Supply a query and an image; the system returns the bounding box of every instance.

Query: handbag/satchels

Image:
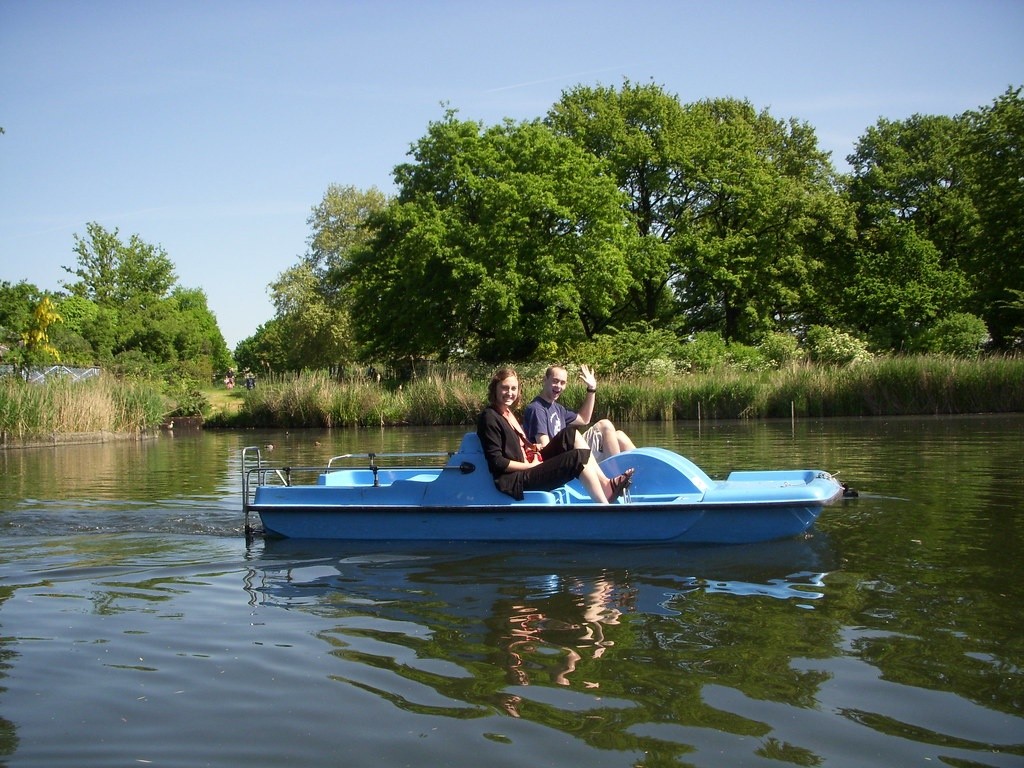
[524,448,542,463]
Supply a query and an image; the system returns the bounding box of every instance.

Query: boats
[239,433,849,544]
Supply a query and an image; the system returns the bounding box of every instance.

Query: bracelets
[586,387,596,392]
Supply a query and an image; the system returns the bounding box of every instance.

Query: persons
[477,368,635,503]
[225,367,235,389]
[523,364,636,459]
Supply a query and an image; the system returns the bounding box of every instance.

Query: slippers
[608,467,634,503]
